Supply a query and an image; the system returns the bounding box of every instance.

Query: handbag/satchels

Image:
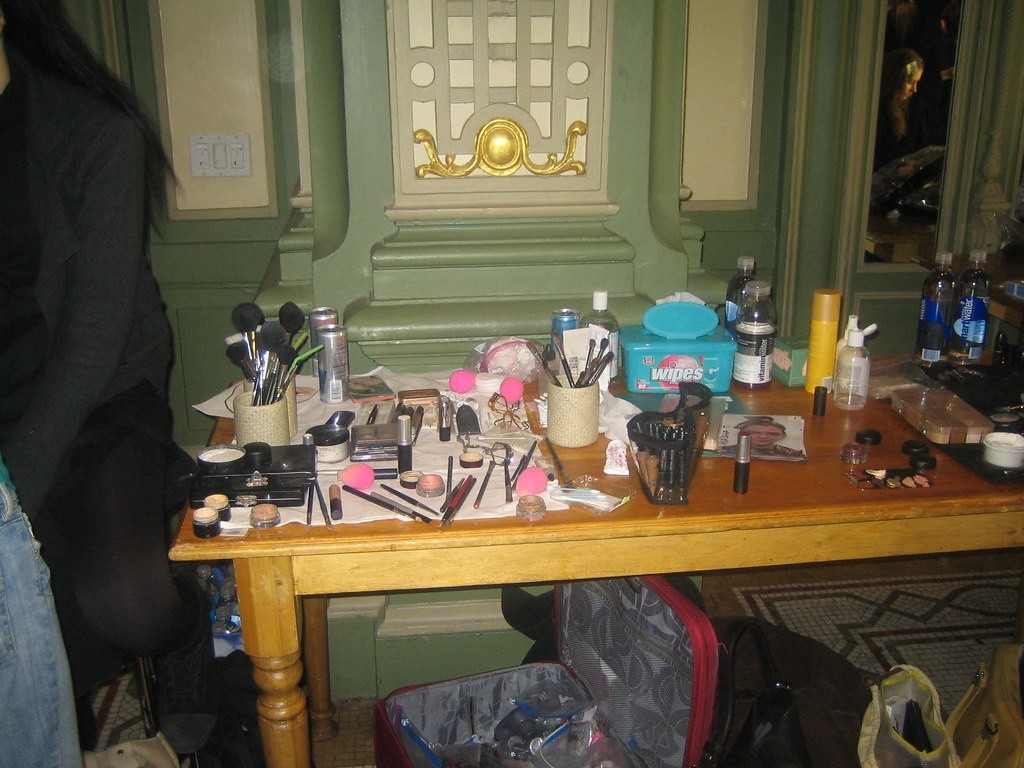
[946,643,1024,768]
[708,586,878,768]
[857,664,961,768]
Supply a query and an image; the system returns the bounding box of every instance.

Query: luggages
[372,575,720,768]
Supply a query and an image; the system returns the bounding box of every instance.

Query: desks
[168,370,1023,768]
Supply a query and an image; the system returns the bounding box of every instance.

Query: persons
[873,48,924,173]
[0,0,217,755]
[721,416,806,458]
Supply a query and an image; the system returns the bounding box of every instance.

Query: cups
[548,375,599,448]
[233,374,298,447]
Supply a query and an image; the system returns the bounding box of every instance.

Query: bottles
[835,315,860,390]
[726,256,776,393]
[833,322,877,411]
[912,248,990,368]
[579,290,620,384]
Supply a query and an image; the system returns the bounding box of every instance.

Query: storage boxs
[984,255,1024,313]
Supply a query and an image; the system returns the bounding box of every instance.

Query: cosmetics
[189,288,1024,538]
[431,676,632,768]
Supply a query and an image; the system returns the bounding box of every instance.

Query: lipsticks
[734,430,752,497]
